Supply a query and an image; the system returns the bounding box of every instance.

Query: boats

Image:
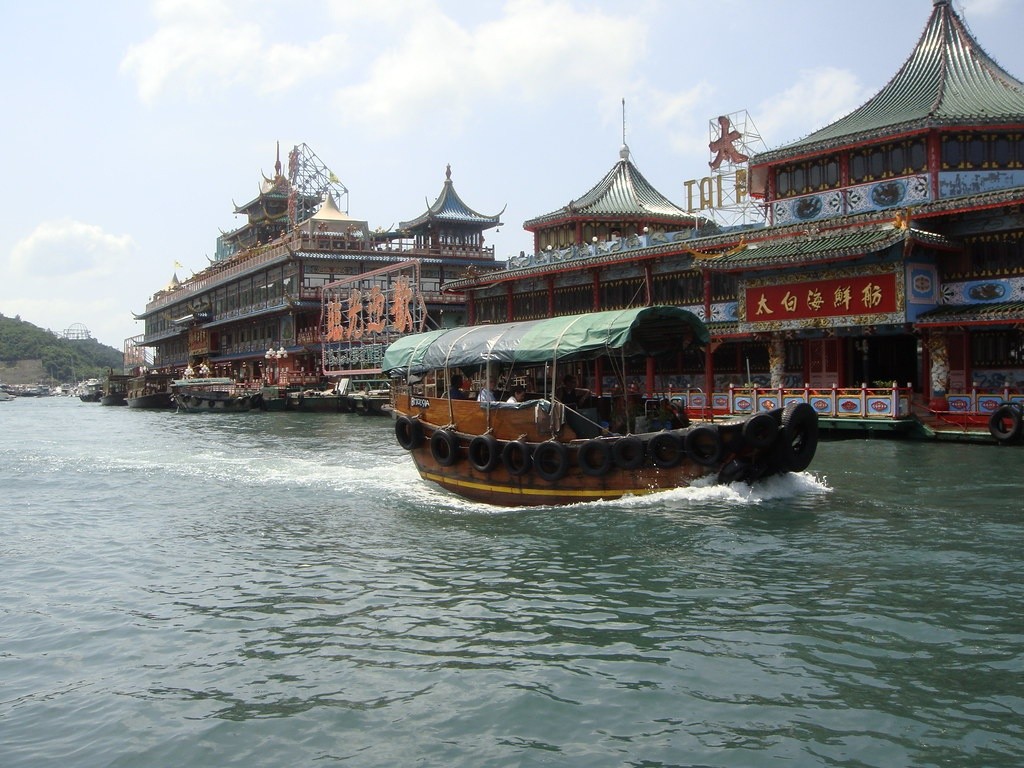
[97,373,139,406]
[381,301,820,508]
[167,375,264,415]
[334,379,392,416]
[283,367,383,412]
[0,358,104,403]
[122,373,180,408]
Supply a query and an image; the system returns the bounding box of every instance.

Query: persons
[450,375,466,399]
[506,386,526,402]
[477,376,496,401]
[557,375,590,410]
[437,374,447,397]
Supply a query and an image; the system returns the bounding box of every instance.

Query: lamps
[592,237,598,242]
[547,245,552,250]
[644,227,648,233]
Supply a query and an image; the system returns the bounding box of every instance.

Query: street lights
[276,346,289,382]
[264,348,277,385]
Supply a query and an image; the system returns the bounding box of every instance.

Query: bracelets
[581,397,588,402]
